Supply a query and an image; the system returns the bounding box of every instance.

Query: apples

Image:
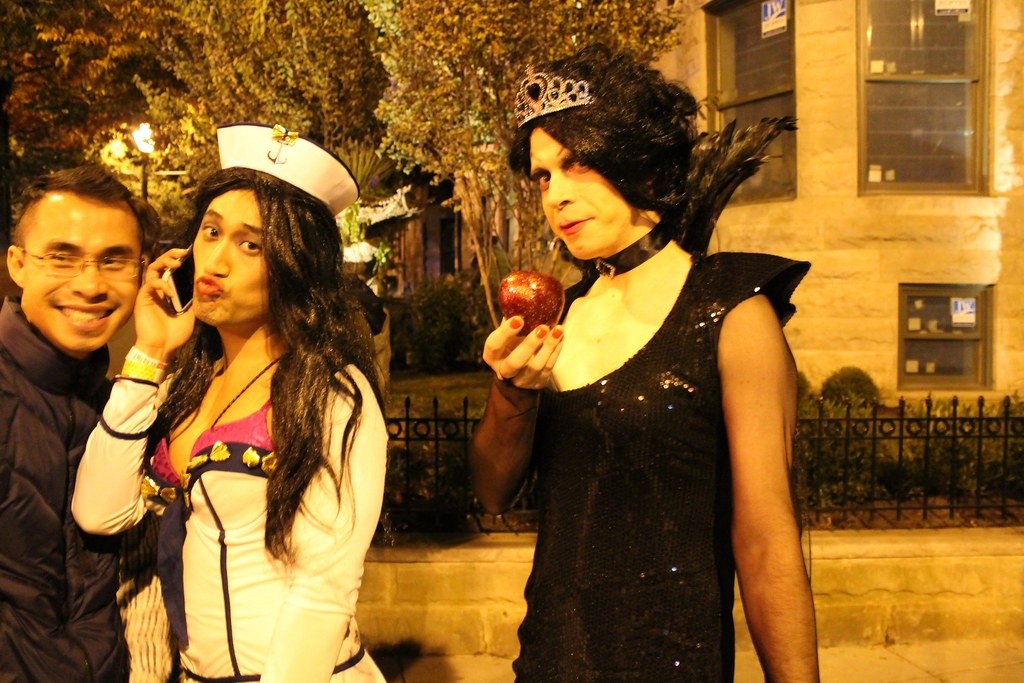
[498,270,565,337]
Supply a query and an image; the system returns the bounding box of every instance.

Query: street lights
[133,123,156,202]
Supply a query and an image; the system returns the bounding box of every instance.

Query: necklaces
[211,352,284,428]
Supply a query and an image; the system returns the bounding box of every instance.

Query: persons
[469,44,820,683]
[71,123,390,683]
[0,164,162,683]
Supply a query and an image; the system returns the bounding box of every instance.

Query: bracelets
[125,348,167,384]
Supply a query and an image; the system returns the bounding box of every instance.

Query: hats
[216,122,360,219]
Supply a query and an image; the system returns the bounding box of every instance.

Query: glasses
[24,251,142,280]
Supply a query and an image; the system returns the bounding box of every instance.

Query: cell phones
[162,244,196,312]
